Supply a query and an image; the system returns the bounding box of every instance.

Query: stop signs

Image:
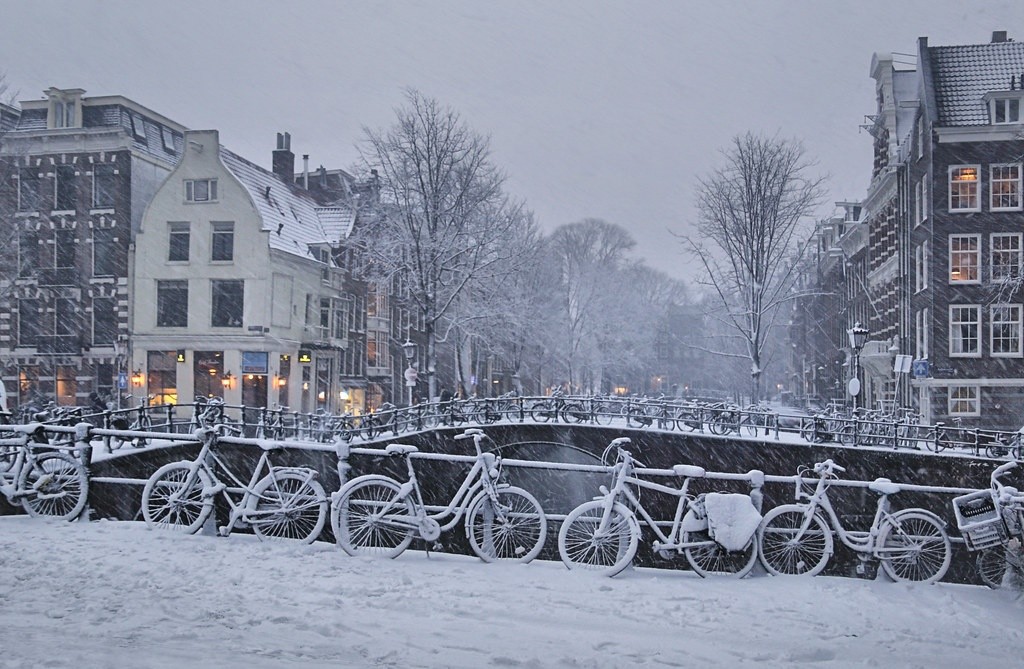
[405,368,418,382]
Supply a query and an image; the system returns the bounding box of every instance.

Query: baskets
[952,489,1010,551]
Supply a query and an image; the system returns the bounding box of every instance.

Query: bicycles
[952,461,1024,589]
[0,424,90,522]
[440,386,1024,460]
[0,391,442,453]
[142,398,333,545]
[757,459,953,585]
[330,429,547,563]
[557,436,760,578]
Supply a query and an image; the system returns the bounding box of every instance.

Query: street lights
[113,334,129,416]
[402,339,418,409]
[847,321,871,447]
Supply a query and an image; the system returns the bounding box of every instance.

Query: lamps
[401,338,418,360]
[846,322,870,352]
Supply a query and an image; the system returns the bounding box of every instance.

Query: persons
[437,381,466,412]
